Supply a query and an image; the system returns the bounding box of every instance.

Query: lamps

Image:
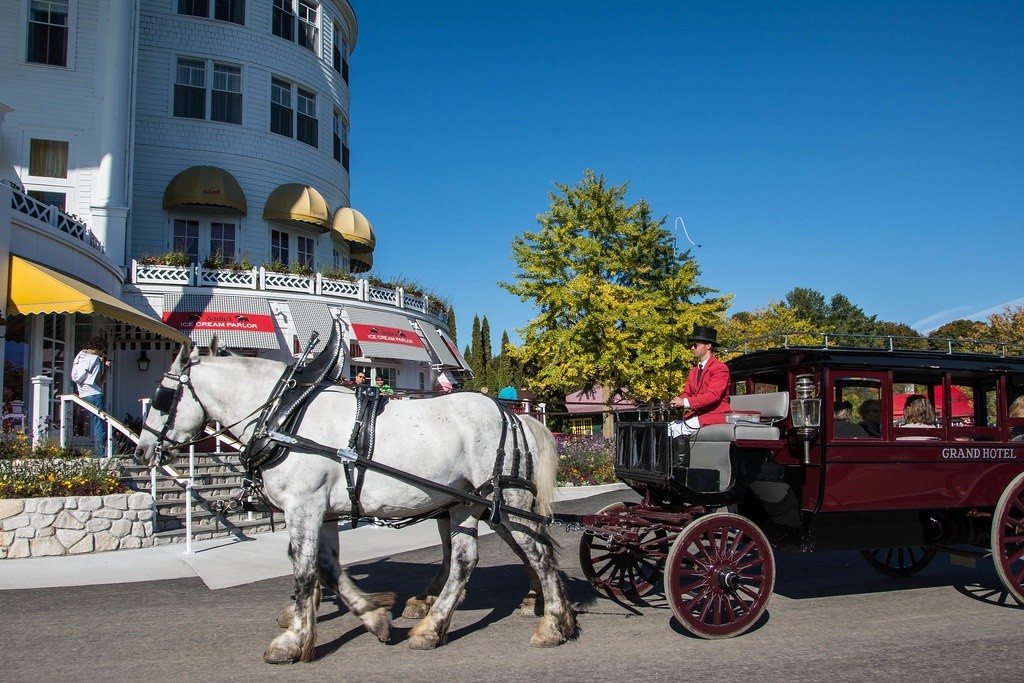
[136,350,151,371]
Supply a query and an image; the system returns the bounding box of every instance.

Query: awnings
[6,253,192,353]
[263,183,333,233]
[350,245,373,273]
[329,206,375,249]
[163,165,248,215]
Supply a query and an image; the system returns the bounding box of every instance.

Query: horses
[134,333,581,667]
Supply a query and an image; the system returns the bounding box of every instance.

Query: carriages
[131,326,1024,663]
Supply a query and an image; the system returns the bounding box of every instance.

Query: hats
[687,327,721,346]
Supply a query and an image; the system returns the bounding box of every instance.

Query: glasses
[359,376,365,379]
[690,343,707,349]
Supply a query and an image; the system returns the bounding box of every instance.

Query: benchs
[678,390,789,490]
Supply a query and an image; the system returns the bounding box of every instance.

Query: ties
[697,364,703,386]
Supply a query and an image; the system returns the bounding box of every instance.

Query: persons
[2,384,25,432]
[373,374,395,395]
[1007,394,1024,442]
[349,372,366,390]
[70,335,112,467]
[895,395,940,440]
[833,398,869,438]
[666,326,732,440]
[857,398,881,437]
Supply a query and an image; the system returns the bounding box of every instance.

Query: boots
[673,434,690,468]
